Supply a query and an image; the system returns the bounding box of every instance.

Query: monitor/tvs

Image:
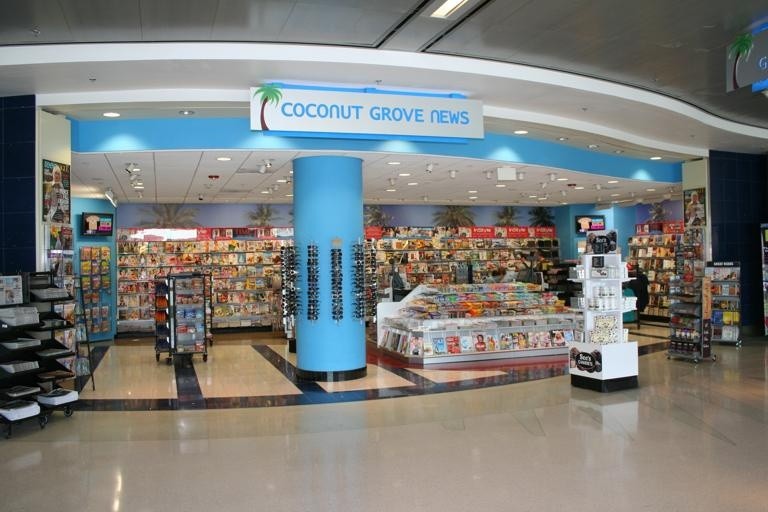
[574,215,606,234]
[82,212,113,236]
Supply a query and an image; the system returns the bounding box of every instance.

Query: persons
[685,190,706,226]
[44,164,66,222]
[482,267,507,284]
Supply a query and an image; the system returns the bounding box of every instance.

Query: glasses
[280,245,298,317]
[307,245,320,320]
[351,243,377,318]
[330,248,344,320]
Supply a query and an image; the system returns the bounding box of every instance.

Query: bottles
[569,264,621,312]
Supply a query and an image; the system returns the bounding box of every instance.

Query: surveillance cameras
[199,195,203,200]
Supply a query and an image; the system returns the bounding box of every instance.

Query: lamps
[125,163,145,200]
[548,173,556,183]
[105,186,118,210]
[259,159,273,175]
[485,171,491,180]
[450,171,457,179]
[518,171,525,182]
[425,163,435,174]
[595,184,601,191]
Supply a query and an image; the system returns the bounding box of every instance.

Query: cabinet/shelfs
[114,225,292,354]
[364,225,581,313]
[572,234,738,382]
[0,272,82,425]
